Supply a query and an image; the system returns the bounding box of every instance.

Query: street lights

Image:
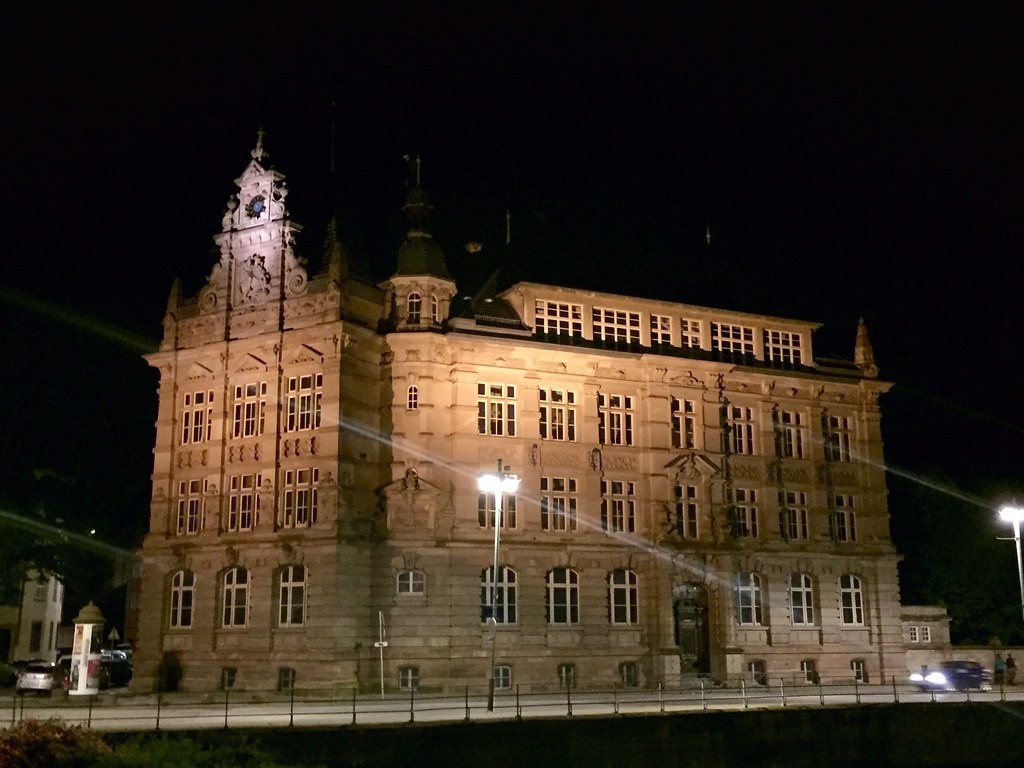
[996,505,1024,625]
[475,474,519,712]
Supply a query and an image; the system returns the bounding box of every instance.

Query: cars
[910,661,992,693]
[0,643,133,688]
[16,662,56,696]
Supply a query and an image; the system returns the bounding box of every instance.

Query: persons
[994,654,1016,684]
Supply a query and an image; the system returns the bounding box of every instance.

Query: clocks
[249,195,266,218]
